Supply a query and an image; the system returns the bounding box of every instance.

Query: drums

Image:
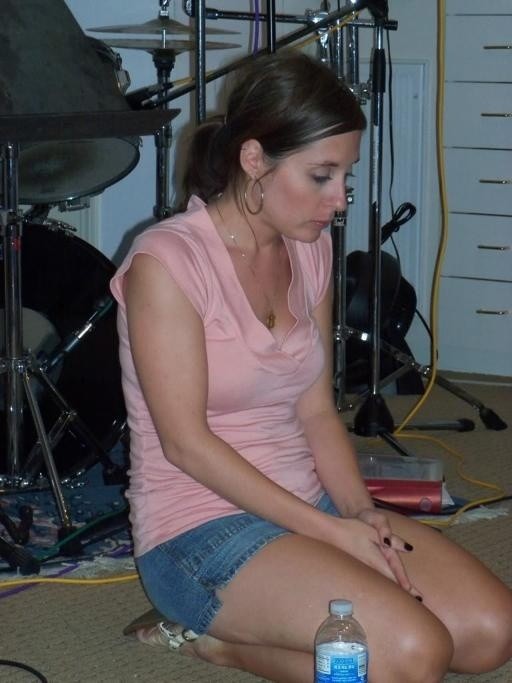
[0,221,127,494]
[0,1,140,205]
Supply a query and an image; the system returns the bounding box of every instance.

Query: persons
[106,40,510,683]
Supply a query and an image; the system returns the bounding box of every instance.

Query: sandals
[122,608,200,651]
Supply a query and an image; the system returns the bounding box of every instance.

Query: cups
[363,478,443,516]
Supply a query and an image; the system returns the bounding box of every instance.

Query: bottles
[314,599,369,683]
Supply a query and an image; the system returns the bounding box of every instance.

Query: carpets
[0,419,509,580]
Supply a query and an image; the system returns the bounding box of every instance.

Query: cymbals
[99,40,243,57]
[85,16,242,34]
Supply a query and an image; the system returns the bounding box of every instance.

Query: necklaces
[215,195,288,329]
